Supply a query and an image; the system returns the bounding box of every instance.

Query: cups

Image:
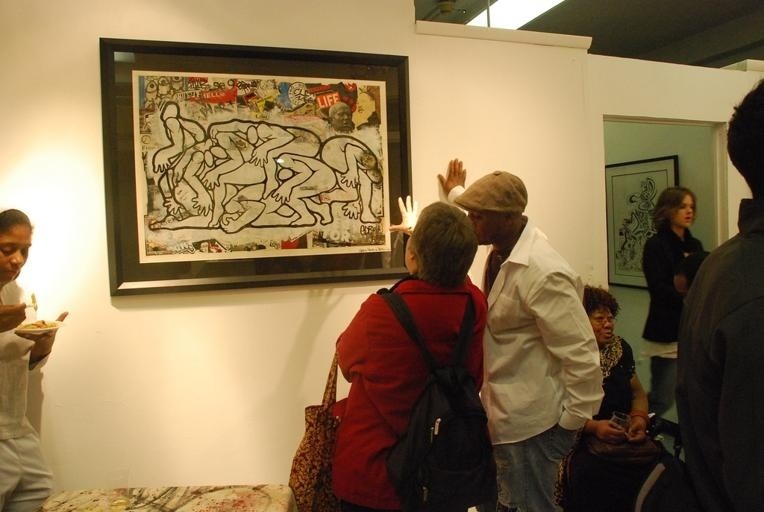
[609,411,633,432]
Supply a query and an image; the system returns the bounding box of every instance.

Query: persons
[575,284,659,468]
[675,80,764,512]
[332,197,489,512]
[641,187,710,416]
[329,102,352,131]
[0,209,69,512]
[437,159,605,512]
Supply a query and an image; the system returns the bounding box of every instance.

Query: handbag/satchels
[633,453,700,512]
[583,411,658,464]
[288,399,343,511]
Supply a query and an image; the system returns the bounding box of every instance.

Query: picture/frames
[605,153,680,290]
[98,36,413,298]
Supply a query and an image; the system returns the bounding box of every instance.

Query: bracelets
[630,410,648,421]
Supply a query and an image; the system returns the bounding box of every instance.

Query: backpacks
[385,292,498,511]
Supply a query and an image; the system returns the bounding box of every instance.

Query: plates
[16,321,63,334]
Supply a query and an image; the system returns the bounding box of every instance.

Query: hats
[454,171,528,214]
